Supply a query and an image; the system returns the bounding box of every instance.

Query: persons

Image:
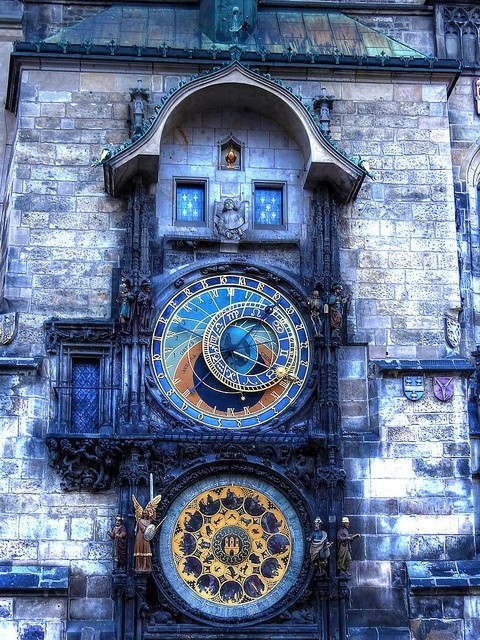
[337,516,354,576]
[217,198,247,240]
[115,278,136,334]
[132,508,155,575]
[135,279,155,336]
[328,284,350,339]
[305,515,333,576]
[106,516,129,573]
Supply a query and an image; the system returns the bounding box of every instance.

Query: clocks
[143,267,316,433]
